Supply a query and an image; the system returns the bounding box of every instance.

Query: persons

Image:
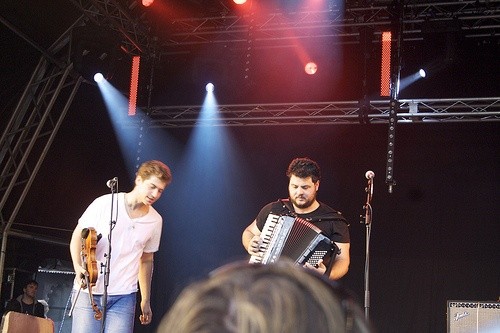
[70,160,172,332]
[0,256,375,333]
[242,158,350,279]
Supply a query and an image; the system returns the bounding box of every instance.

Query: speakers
[446,300,500,333]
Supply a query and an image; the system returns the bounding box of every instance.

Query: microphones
[106,177,119,188]
[365,170,375,179]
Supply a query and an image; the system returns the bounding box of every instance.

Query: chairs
[1,311,54,333]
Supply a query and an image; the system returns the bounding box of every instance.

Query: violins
[80,227,103,321]
